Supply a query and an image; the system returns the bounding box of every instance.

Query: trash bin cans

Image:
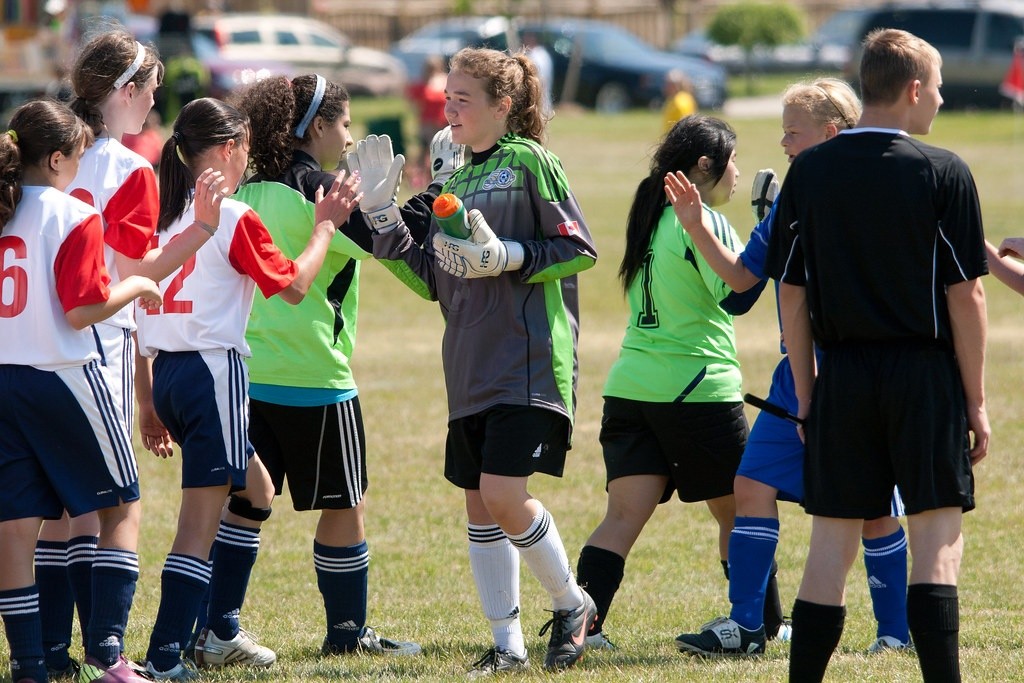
[366,113,406,161]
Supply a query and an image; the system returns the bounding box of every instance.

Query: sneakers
[144,658,198,683]
[674,616,767,660]
[537,587,598,672]
[585,629,620,652]
[203,627,275,667]
[182,632,198,670]
[869,633,913,655]
[316,624,419,663]
[774,614,794,641]
[46,655,81,682]
[194,628,209,669]
[77,655,153,683]
[467,645,530,680]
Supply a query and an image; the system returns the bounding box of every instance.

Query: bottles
[432,194,472,240]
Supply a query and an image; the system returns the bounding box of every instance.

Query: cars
[385,15,511,78]
[62,5,294,119]
[671,4,873,77]
[194,10,406,99]
[441,15,730,115]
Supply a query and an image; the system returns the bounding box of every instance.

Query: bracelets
[195,219,217,236]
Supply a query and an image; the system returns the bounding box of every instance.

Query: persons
[763,27,991,683]
[346,48,598,672]
[200,74,469,669]
[663,77,914,660]
[0,98,164,683]
[11,28,165,680]
[524,27,552,124]
[576,117,792,652]
[134,98,367,683]
[985,237,1024,296]
[663,72,695,138]
[405,54,454,187]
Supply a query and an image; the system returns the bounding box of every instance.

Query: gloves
[345,134,415,253]
[432,209,525,278]
[749,169,779,225]
[428,125,466,187]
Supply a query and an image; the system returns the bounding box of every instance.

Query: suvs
[840,0,1024,111]
